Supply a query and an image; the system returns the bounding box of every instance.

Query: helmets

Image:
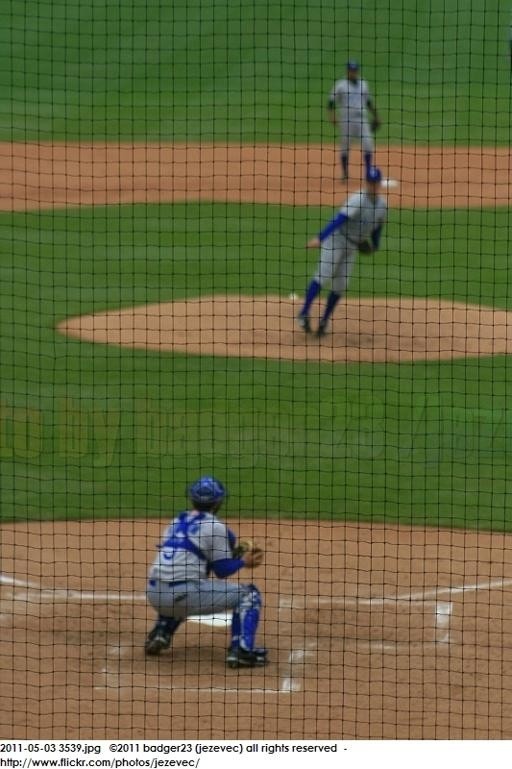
[191,475,225,503]
[366,166,381,181]
[347,62,358,71]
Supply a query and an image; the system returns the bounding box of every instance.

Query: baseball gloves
[235,536,266,567]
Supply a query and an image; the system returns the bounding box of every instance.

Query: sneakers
[317,320,327,332]
[226,647,268,666]
[298,312,310,330]
[145,630,171,654]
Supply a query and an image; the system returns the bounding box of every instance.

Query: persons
[327,62,381,182]
[144,476,269,669]
[297,167,389,337]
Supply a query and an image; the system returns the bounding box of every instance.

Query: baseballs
[290,293,299,300]
